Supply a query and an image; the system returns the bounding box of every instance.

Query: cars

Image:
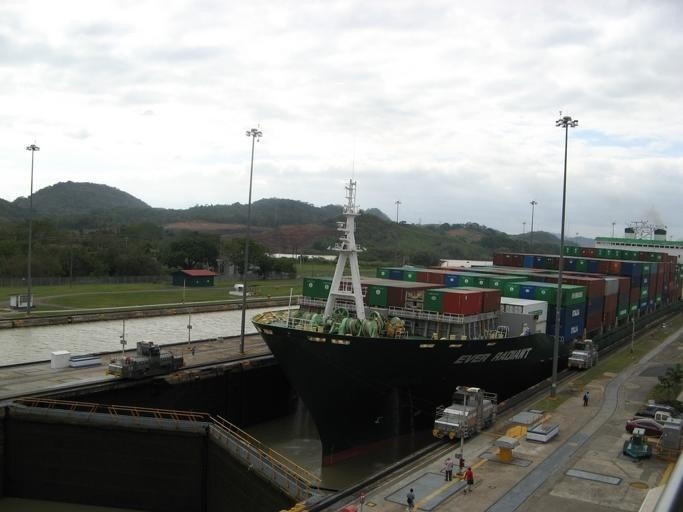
[625,418,663,437]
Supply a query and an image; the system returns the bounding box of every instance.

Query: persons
[445,458,453,481]
[406,488,415,512]
[463,467,473,493]
[520,323,529,336]
[583,392,591,406]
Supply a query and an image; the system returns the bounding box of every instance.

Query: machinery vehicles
[106,340,187,381]
[429,384,497,440]
[568,337,598,369]
[622,428,652,460]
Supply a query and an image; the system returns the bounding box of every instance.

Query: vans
[638,404,678,417]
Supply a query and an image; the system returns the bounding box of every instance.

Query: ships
[252,179,683,464]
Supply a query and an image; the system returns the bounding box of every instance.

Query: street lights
[394,200,405,224]
[549,113,579,400]
[524,195,617,242]
[240,128,262,355]
[26,145,39,316]
[359,492,365,512]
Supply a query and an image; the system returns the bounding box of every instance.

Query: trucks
[629,411,682,429]
